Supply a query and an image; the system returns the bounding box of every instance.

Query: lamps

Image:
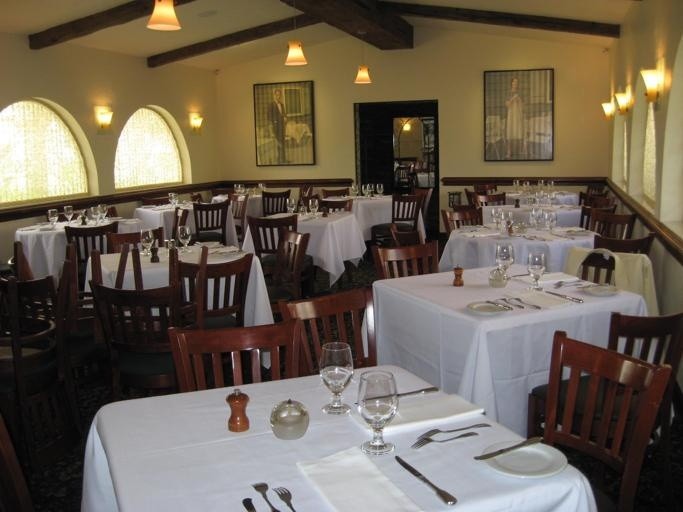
[598,69,665,120]
[354,40,372,85]
[284,2,307,66]
[144,1,181,32]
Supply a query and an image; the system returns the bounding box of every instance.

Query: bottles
[452,264,464,286]
[487,266,508,286]
[270,396,309,441]
[224,388,249,432]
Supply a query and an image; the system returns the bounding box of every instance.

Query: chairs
[396,166,411,194]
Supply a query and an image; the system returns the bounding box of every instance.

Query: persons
[503,75,525,159]
[265,87,294,164]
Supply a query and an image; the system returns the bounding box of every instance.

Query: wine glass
[487,178,557,238]
[47,183,385,262]
[527,254,546,292]
[496,244,514,280]
[359,369,400,456]
[319,342,354,416]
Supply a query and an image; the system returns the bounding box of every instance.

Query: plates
[584,284,621,297]
[481,440,567,478]
[466,301,501,315]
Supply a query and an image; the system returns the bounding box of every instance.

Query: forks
[253,480,281,512]
[416,422,491,440]
[411,432,478,450]
[271,486,296,512]
[501,296,543,311]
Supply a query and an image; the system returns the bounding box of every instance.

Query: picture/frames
[252,80,315,167]
[482,67,553,162]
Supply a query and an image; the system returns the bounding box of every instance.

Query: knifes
[394,455,456,504]
[487,300,511,310]
[242,498,255,512]
[354,386,438,404]
[545,291,583,303]
[473,436,542,460]
